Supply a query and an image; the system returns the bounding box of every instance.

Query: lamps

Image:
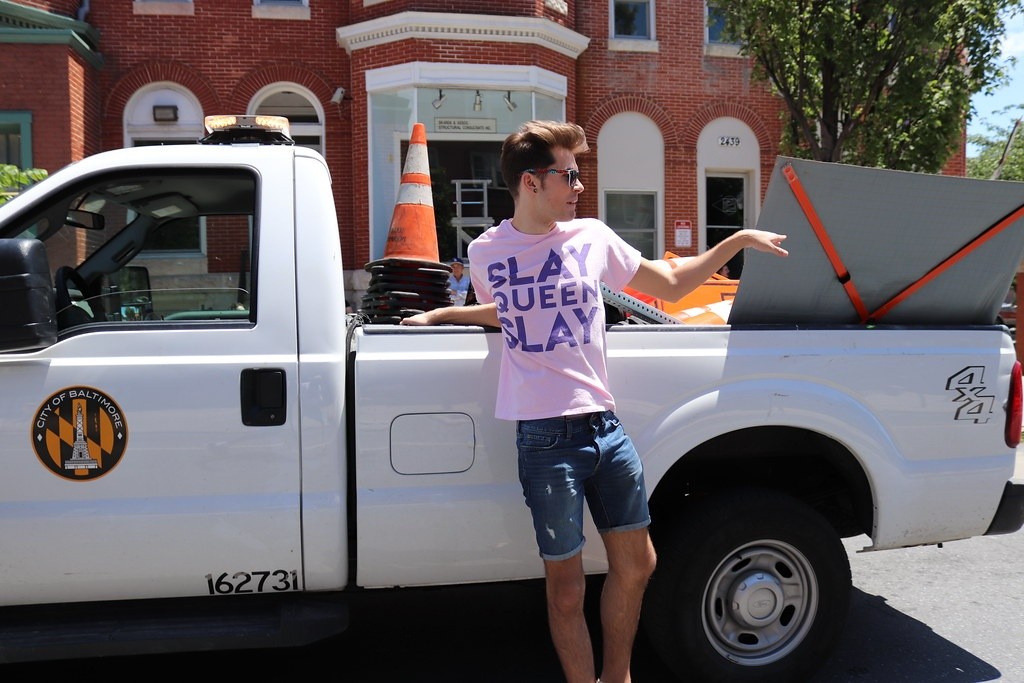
[504,90,520,110]
[472,89,483,112]
[430,89,449,110]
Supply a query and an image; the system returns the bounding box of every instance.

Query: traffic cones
[358,120,454,335]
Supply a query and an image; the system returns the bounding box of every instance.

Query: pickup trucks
[0,114,1021,682]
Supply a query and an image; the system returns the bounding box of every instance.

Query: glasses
[517,167,580,188]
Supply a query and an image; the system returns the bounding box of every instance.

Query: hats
[451,258,465,266]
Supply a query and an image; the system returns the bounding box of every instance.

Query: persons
[447,257,470,298]
[399,121,790,683]
